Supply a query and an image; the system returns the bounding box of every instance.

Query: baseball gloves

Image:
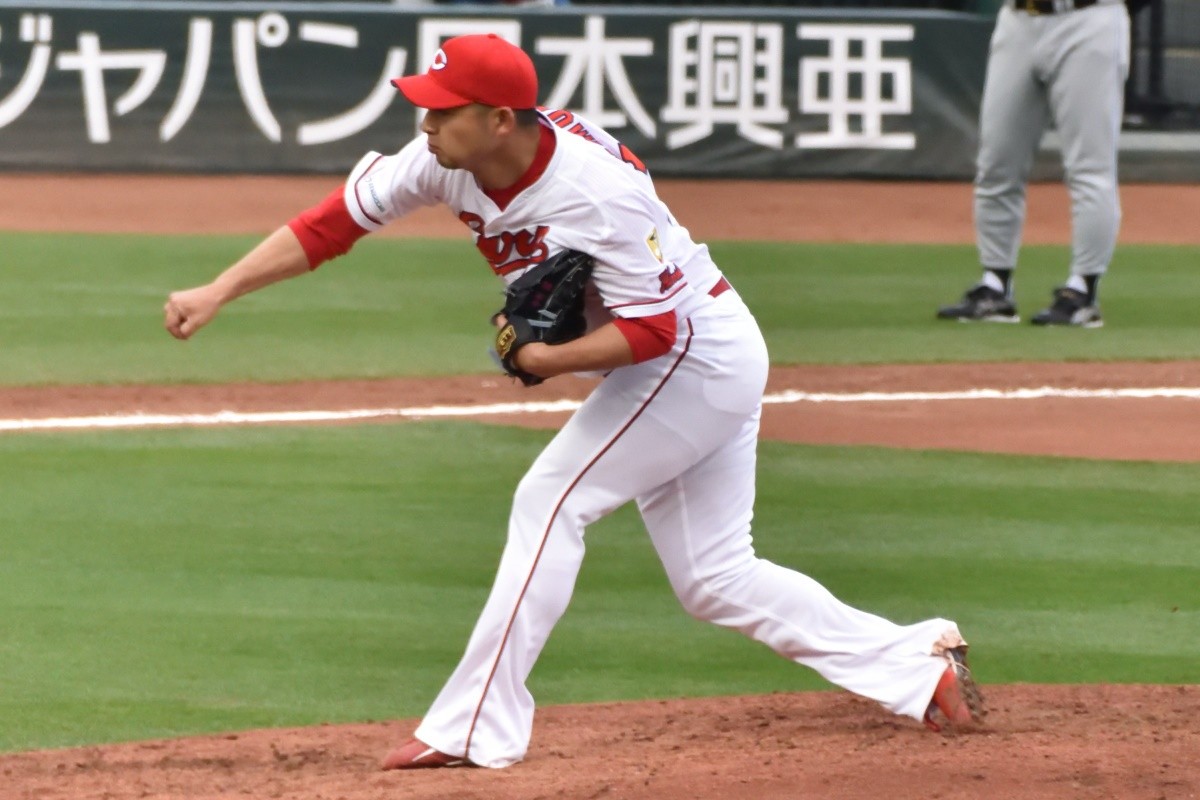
[490,248,597,389]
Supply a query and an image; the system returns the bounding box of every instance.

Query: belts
[1016,0,1094,15]
[709,276,731,298]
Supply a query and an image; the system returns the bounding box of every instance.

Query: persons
[162,32,988,770]
[936,0,1132,327]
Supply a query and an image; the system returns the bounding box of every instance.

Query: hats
[393,34,538,111]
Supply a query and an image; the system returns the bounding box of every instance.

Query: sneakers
[1028,288,1103,328]
[934,287,1020,323]
[381,736,469,771]
[925,644,986,732]
